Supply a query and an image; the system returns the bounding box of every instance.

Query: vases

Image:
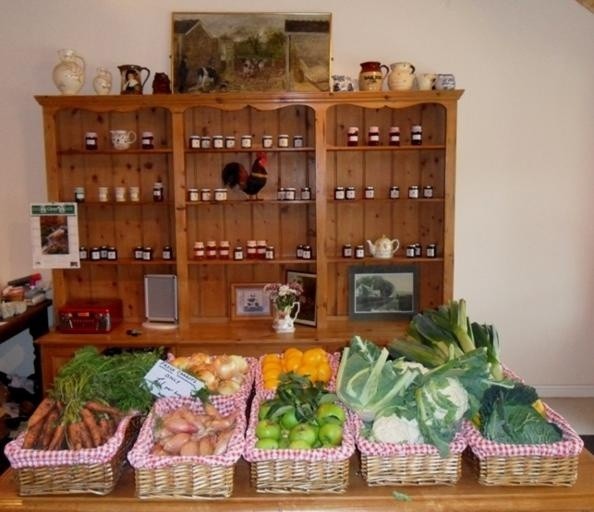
[272,302,301,332]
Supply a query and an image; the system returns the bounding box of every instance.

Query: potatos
[149,401,239,459]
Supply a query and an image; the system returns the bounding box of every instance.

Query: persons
[123,70,138,90]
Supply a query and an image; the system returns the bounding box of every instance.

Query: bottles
[342,244,364,258]
[348,124,423,147]
[335,185,433,200]
[277,187,310,200]
[161,245,172,260]
[189,134,305,148]
[193,240,313,260]
[405,243,436,258]
[135,245,153,261]
[74,186,86,203]
[153,182,164,201]
[80,245,118,261]
[187,187,228,201]
[84,131,98,150]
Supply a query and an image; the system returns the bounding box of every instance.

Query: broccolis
[335,333,488,458]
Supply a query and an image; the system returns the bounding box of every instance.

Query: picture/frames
[285,268,316,328]
[144,274,178,322]
[170,11,333,95]
[230,282,271,319]
[348,264,419,321]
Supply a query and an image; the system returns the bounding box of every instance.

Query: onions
[169,353,250,397]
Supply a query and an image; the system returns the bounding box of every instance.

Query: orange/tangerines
[260,346,335,391]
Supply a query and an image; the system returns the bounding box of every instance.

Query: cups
[109,129,137,150]
[117,65,150,95]
[357,61,442,92]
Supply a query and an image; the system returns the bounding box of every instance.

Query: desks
[0,441,594,511]
[1,298,54,405]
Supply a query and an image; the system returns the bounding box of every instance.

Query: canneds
[342,242,366,259]
[276,186,312,202]
[85,131,99,151]
[72,182,165,205]
[295,243,312,259]
[133,245,173,262]
[346,125,424,148]
[404,241,437,258]
[334,185,435,201]
[79,245,119,262]
[189,133,305,150]
[187,187,228,204]
[191,238,275,262]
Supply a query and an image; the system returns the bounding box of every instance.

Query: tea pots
[366,234,400,259]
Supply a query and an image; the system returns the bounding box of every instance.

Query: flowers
[260,280,304,311]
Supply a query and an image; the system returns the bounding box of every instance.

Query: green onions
[384,297,505,391]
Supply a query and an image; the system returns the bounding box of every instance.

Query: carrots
[21,398,125,451]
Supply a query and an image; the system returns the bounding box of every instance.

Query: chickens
[221,152,267,201]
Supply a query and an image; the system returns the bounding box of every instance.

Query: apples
[255,399,345,449]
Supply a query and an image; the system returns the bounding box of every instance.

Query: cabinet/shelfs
[34,90,462,402]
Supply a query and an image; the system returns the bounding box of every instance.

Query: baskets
[356,415,466,487]
[4,409,141,495]
[130,352,354,501]
[466,365,583,487]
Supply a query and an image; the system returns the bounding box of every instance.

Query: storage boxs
[57,298,124,334]
